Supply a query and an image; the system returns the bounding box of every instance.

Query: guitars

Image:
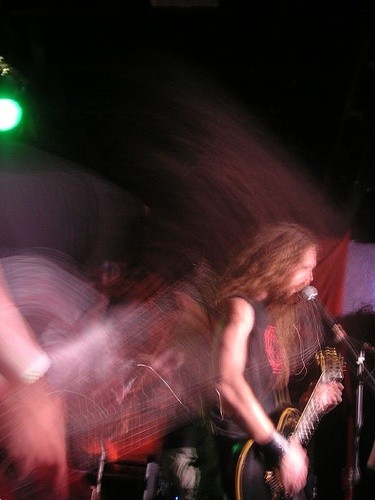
[221,345,346,500]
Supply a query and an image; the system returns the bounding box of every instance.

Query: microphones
[303,286,347,343]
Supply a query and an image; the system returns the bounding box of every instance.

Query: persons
[202,223,324,500]
